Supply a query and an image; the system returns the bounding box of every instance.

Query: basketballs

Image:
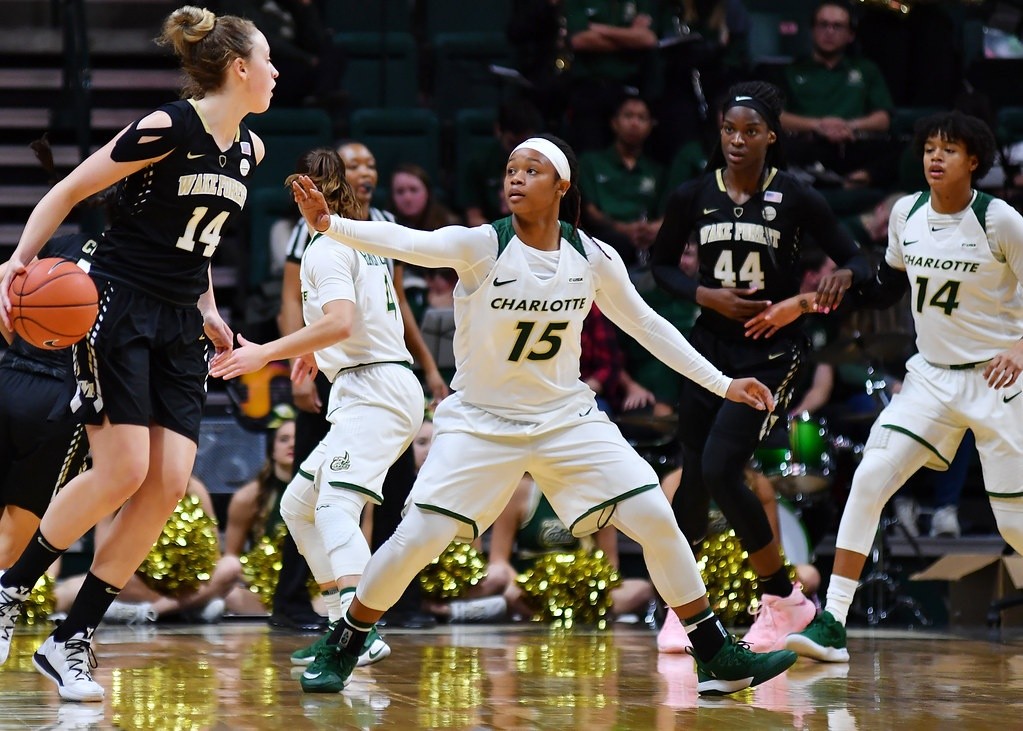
[8,257,99,350]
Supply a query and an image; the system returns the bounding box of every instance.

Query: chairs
[245,0,514,276]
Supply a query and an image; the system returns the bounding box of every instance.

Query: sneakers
[32,619,104,701]
[269,611,329,631]
[181,597,226,622]
[0,569,30,666]
[739,581,815,653]
[684,635,798,697]
[291,662,375,682]
[656,605,694,653]
[102,599,157,630]
[355,625,391,668]
[785,610,850,662]
[290,619,338,666]
[451,596,507,624]
[300,639,358,694]
[379,608,436,628]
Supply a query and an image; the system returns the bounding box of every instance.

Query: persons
[1,178,120,577]
[38,0,1023,622]
[279,141,447,630]
[0,2,280,700]
[294,135,793,692]
[792,109,1023,663]
[647,83,862,652]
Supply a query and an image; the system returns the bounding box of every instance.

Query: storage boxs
[907,551,1023,628]
